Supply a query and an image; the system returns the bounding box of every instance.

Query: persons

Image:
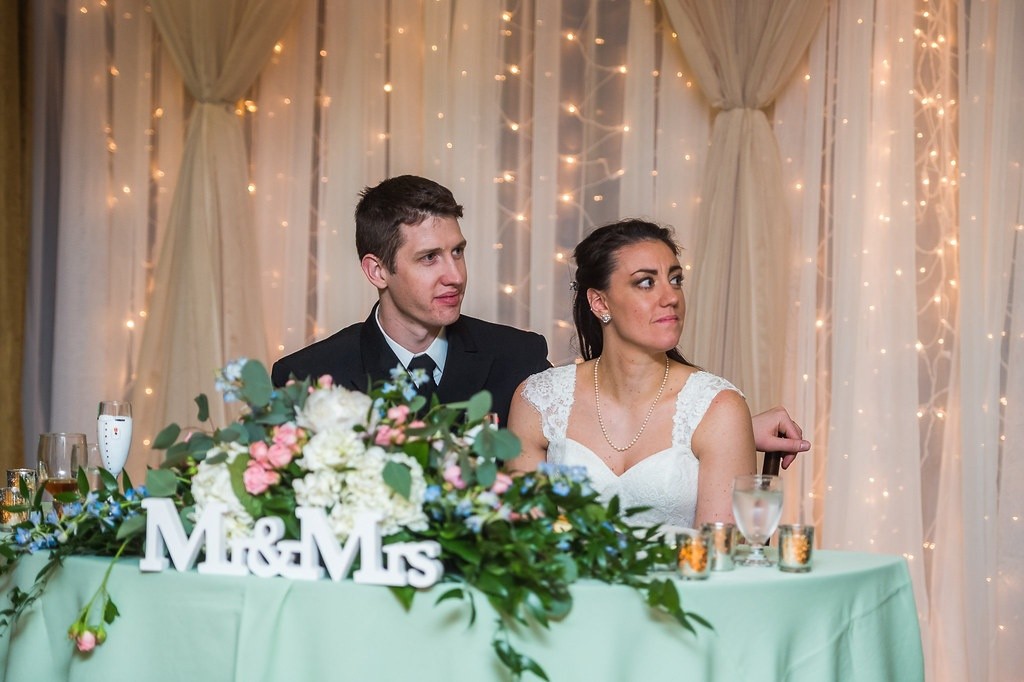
[502,220,760,539]
[270,177,812,470]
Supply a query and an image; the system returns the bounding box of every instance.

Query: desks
[0,500,924,682]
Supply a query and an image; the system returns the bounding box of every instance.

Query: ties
[408,353,438,420]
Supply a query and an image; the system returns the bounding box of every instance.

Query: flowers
[0,375,715,682]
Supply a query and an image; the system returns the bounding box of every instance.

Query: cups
[779,524,814,572]
[97,401,133,479]
[7,469,36,491]
[702,522,736,571]
[676,532,713,580]
[0,486,33,528]
[37,433,89,504]
[72,444,103,493]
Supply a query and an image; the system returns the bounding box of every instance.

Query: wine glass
[732,476,783,567]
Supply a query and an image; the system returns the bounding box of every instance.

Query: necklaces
[593,354,670,453]
